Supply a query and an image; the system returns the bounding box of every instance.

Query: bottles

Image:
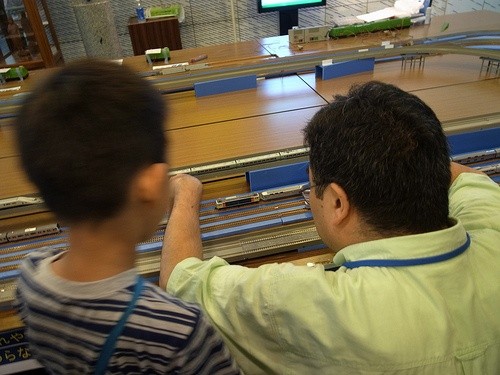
[136,0,146,24]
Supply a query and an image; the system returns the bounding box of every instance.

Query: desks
[0,10,500,331]
[128,15,182,56]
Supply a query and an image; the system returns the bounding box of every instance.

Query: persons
[12,56,238,375]
[159,80,500,375]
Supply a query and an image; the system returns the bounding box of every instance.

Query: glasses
[300,184,319,206]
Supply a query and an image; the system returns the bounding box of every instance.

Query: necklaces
[342,232,471,269]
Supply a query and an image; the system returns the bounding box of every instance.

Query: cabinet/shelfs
[0,0,65,71]
